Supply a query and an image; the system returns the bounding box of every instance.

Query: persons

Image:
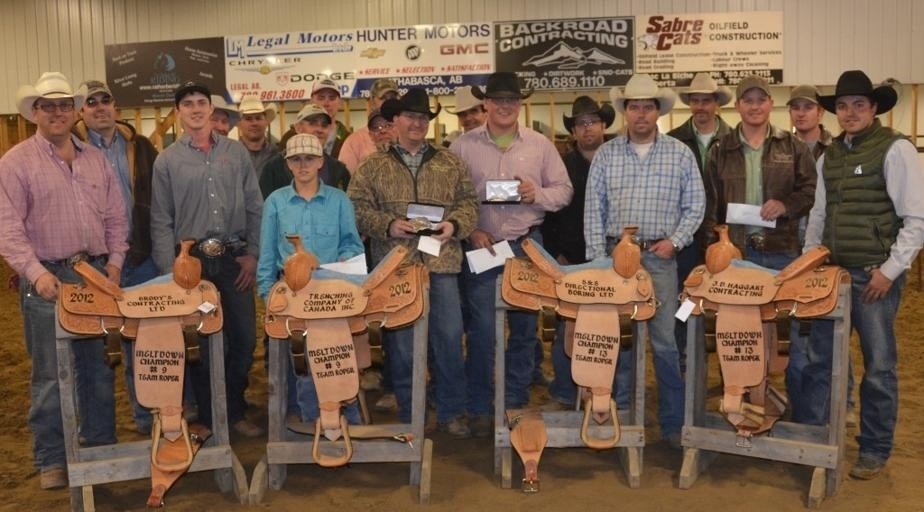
[803,72,922,480]
[78,79,158,450]
[146,75,733,457]
[0,70,130,493]
[703,76,805,428]
[781,86,862,431]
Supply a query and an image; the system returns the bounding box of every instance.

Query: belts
[495,224,540,245]
[726,234,789,251]
[606,236,661,250]
[51,250,106,268]
[189,237,240,259]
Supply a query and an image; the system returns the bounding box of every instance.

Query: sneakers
[374,393,403,413]
[660,424,683,450]
[436,411,495,438]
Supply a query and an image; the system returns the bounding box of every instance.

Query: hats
[446,85,484,118]
[615,73,677,114]
[16,69,114,126]
[284,133,323,160]
[472,70,535,100]
[819,70,898,114]
[366,79,401,128]
[734,76,773,100]
[294,104,333,126]
[310,79,341,97]
[174,81,279,127]
[562,95,615,133]
[381,88,442,121]
[785,85,822,106]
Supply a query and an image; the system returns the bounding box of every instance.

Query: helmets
[679,73,731,108]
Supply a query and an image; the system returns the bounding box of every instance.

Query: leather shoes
[848,456,886,480]
[231,418,266,437]
[40,467,66,490]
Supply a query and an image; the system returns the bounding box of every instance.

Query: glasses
[33,102,76,114]
[573,117,602,129]
[370,123,392,132]
[85,95,112,107]
